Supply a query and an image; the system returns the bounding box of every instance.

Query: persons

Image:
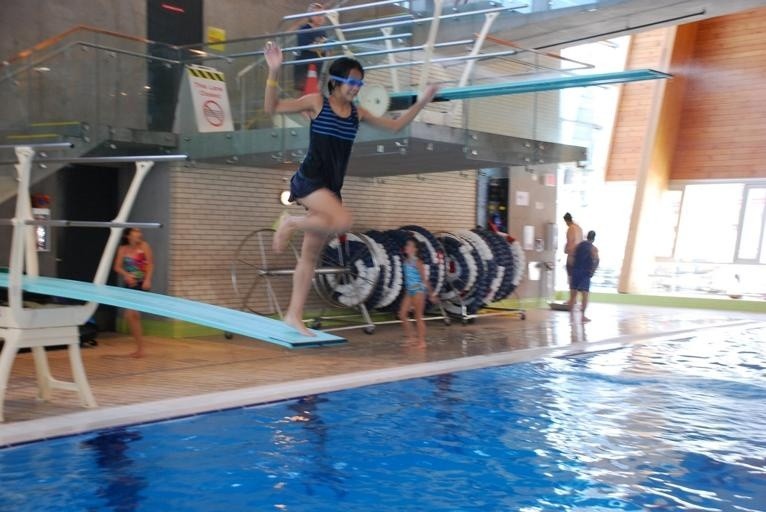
[564,229,601,323]
[261,37,441,340]
[561,211,584,304]
[286,391,352,512]
[113,227,154,358]
[397,236,440,350]
[291,1,335,123]
[429,373,462,446]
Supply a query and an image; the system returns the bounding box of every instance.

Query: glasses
[330,75,364,87]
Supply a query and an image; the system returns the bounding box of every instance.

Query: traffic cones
[301,65,321,101]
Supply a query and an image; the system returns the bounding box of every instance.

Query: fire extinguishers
[35,214,46,250]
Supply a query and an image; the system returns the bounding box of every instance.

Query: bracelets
[264,79,279,88]
